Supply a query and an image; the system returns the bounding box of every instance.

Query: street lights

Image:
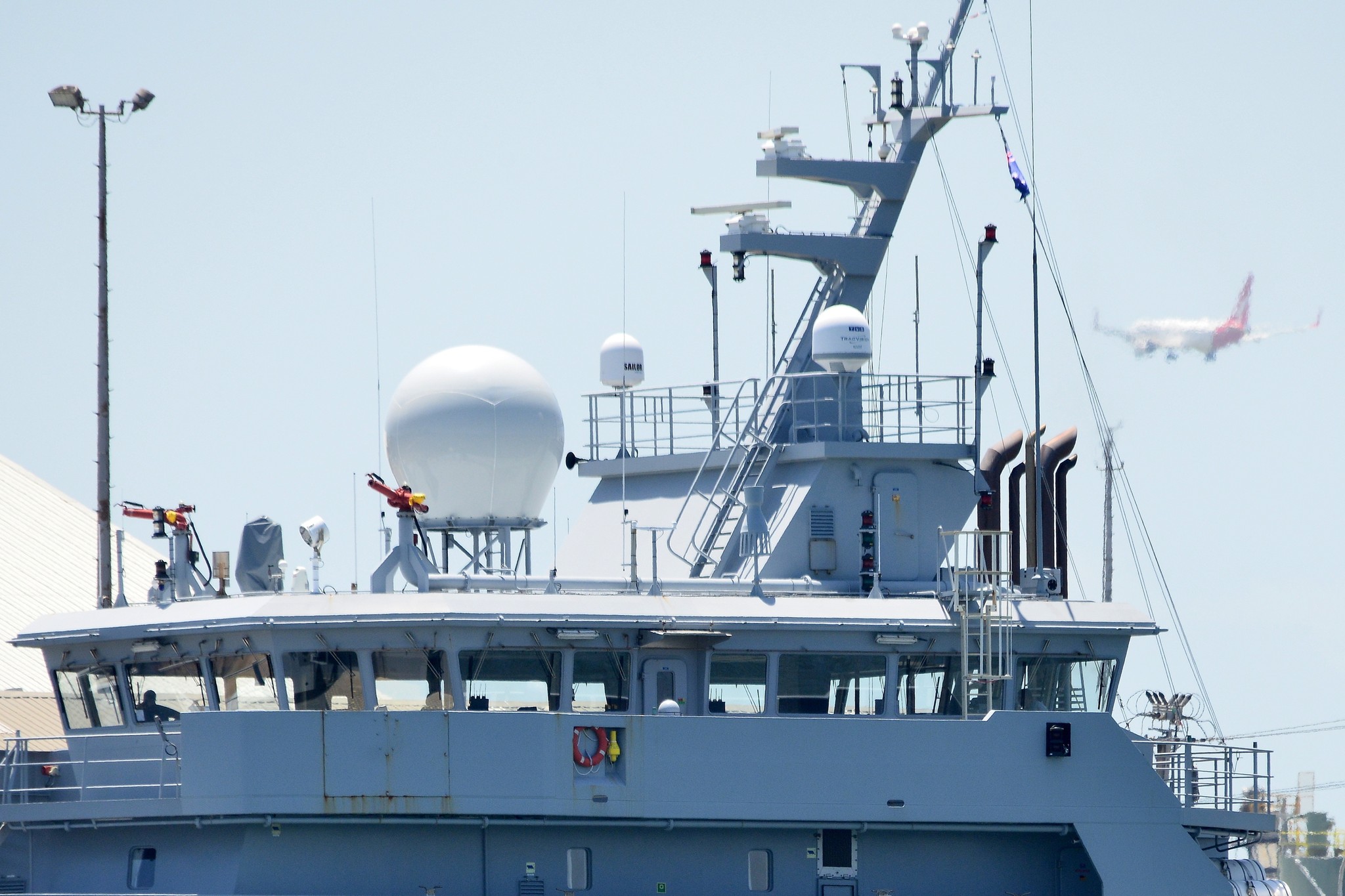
[48,85,156,614]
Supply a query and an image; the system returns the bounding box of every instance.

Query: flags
[1004,141,1030,201]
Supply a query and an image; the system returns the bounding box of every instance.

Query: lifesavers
[573,726,608,768]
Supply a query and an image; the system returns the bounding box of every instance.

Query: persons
[137,690,180,721]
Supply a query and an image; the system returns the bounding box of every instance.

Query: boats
[2,2,1296,896]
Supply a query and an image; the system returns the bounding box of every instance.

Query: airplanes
[1091,272,1322,366]
